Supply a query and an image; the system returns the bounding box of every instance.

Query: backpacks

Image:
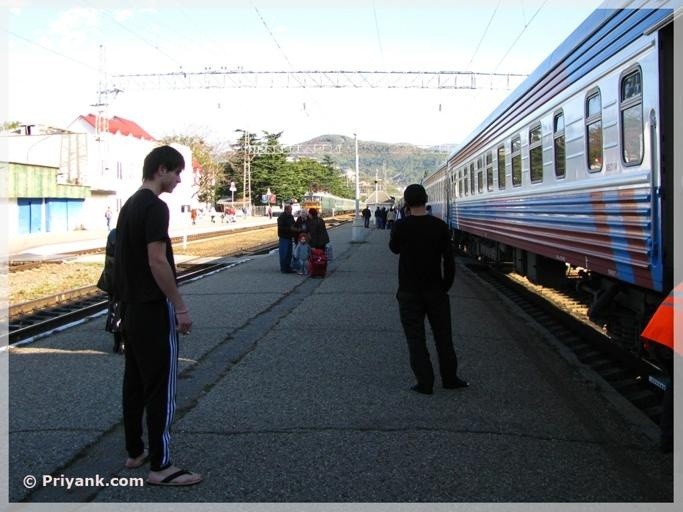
[307,249,327,278]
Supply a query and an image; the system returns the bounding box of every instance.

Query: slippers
[146,468,204,486]
[126,446,150,469]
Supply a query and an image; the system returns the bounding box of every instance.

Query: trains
[392,0,681,405]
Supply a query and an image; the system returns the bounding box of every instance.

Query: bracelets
[174,308,189,315]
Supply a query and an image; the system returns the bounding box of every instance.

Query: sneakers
[410,383,433,395]
[299,272,309,275]
[443,381,470,389]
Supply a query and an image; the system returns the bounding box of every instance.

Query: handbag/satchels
[325,244,333,261]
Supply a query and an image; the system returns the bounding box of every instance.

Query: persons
[104,206,112,232]
[113,143,205,488]
[360,206,433,230]
[190,208,197,225]
[639,282,681,454]
[93,227,127,356]
[268,207,272,219]
[385,182,470,396]
[272,204,330,277]
[206,205,237,224]
